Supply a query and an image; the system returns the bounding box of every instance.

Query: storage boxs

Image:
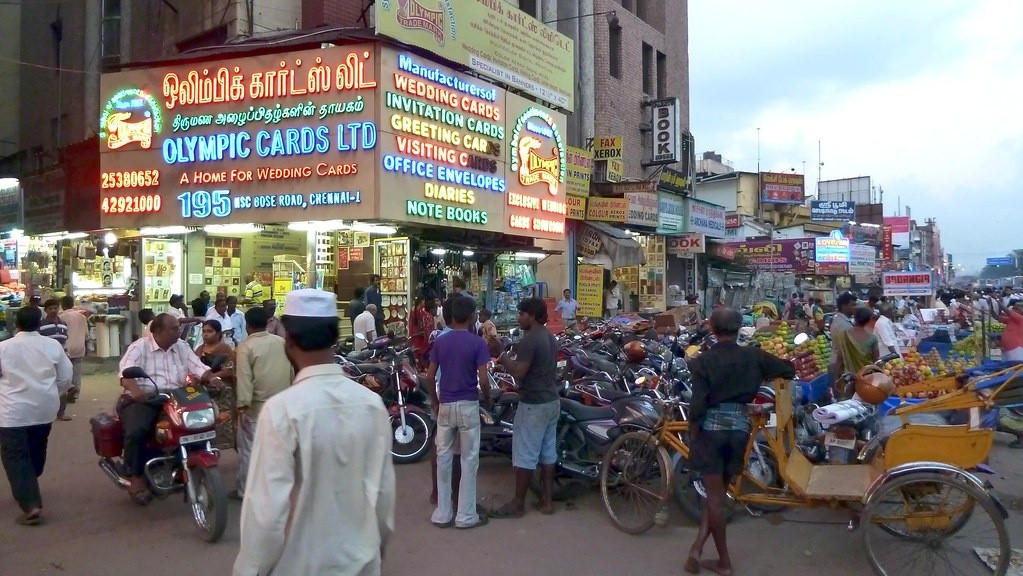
[90,413,125,458]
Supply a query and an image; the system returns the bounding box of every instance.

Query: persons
[682,307,796,576]
[897,286,1019,318]
[138,291,248,452]
[486,298,564,518]
[347,275,387,351]
[262,299,287,339]
[986,293,1023,360]
[406,278,499,529]
[241,274,263,304]
[227,307,295,500]
[553,289,582,335]
[790,290,904,401]
[28,295,91,421]
[117,314,225,508]
[0,306,73,524]
[605,279,620,318]
[103,261,111,285]
[233,289,398,576]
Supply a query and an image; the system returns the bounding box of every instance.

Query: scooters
[332,315,901,525]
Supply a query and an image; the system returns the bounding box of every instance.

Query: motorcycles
[89,355,228,543]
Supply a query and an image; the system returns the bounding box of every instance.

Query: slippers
[473,515,488,526]
[490,507,514,517]
[436,522,450,527]
[15,514,43,524]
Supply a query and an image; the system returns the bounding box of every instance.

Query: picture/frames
[337,228,354,247]
[354,231,371,248]
[339,247,350,270]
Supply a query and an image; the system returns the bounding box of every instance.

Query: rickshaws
[600,365,1023,576]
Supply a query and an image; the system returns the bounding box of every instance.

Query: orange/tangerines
[754,320,832,372]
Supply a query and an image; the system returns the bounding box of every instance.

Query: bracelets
[213,376,221,380]
[995,297,1000,301]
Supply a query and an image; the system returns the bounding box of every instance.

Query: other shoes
[1010,438,1023,449]
[58,414,71,421]
[132,486,150,505]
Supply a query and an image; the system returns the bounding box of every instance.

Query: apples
[947,323,1007,374]
[883,345,946,399]
[772,337,821,382]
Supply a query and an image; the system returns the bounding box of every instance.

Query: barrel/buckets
[879,396,928,420]
[958,329,1004,348]
[920,339,952,374]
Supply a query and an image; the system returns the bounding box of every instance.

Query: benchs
[878,395,997,446]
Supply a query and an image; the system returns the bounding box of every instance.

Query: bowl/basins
[920,309,938,321]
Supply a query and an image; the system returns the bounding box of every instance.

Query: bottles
[935,310,948,324]
[975,319,981,330]
[757,318,769,329]
[912,336,917,346]
[963,315,974,332]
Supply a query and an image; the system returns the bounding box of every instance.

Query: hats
[170,294,183,306]
[262,299,277,309]
[284,289,337,317]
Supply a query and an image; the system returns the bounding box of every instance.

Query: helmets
[855,364,896,404]
[624,341,646,361]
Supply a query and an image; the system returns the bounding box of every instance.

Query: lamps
[513,251,546,259]
[545,11,621,27]
